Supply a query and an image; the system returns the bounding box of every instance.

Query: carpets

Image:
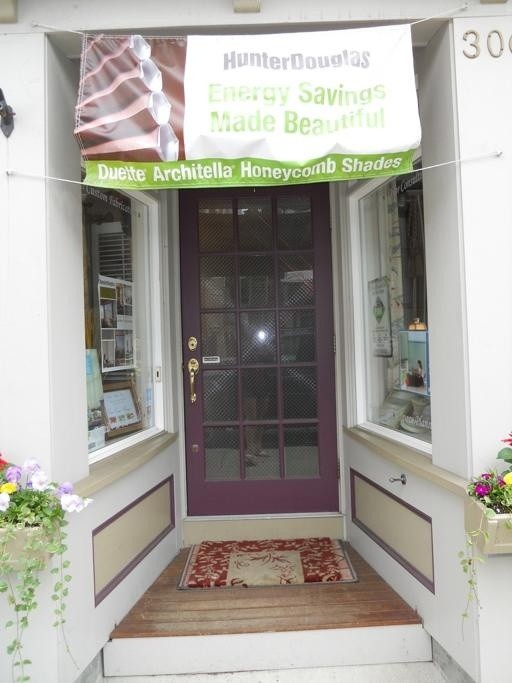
[177,536,359,591]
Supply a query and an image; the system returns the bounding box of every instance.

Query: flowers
[0,452,93,683]
[457,431,512,643]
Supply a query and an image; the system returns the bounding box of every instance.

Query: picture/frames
[99,381,145,440]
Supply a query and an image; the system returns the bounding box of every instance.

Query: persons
[232,323,279,467]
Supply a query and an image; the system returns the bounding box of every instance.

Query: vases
[464,490,512,554]
[0,522,62,572]
